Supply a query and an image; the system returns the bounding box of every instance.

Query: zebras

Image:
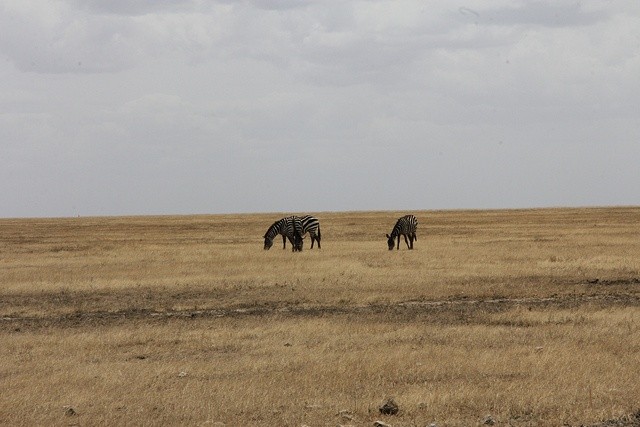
[385,214,418,250]
[262,214,322,249]
[285,215,306,251]
